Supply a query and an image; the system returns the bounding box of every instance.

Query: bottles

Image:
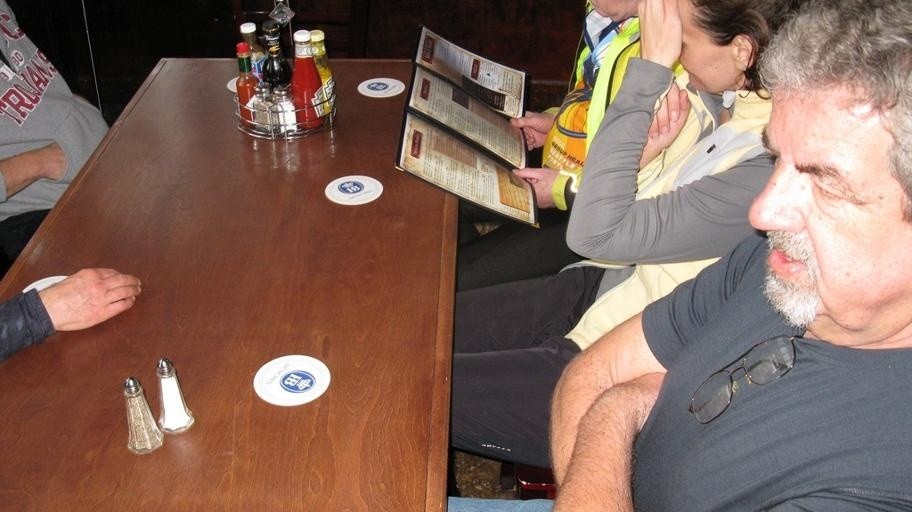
[123,377,163,456]
[254,82,279,134]
[156,356,193,435]
[273,86,298,134]
[261,21,296,107]
[291,29,325,128]
[234,43,260,128]
[310,31,335,116]
[239,22,265,83]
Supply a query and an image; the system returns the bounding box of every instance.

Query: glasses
[687,332,805,425]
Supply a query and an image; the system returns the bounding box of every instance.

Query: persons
[1,1,112,278]
[449,2,803,470]
[446,1,911,512]
[0,265,145,365]
[457,2,691,288]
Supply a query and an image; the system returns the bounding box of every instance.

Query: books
[395,22,542,231]
